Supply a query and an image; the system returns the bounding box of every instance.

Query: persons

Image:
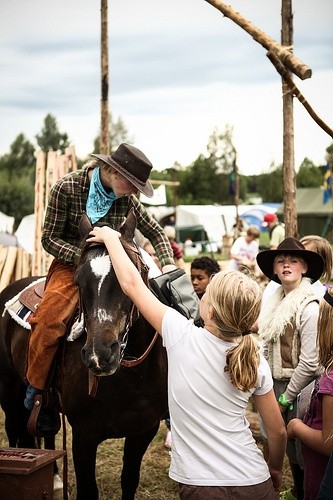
[143,210,332,449]
[21,143,178,435]
[85,225,287,500]
[254,236,325,500]
[287,289,333,500]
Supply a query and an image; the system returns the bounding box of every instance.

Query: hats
[262,213,277,227]
[256,237,327,285]
[88,141,155,199]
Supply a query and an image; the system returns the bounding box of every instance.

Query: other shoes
[23,385,46,411]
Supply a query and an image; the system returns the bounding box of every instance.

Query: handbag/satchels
[148,268,199,322]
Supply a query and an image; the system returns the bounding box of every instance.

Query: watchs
[279,394,294,411]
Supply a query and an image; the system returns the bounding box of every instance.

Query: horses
[0,207,169,500]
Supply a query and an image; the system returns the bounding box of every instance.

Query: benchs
[176,228,219,261]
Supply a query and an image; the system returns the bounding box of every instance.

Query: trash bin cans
[0,446,67,500]
